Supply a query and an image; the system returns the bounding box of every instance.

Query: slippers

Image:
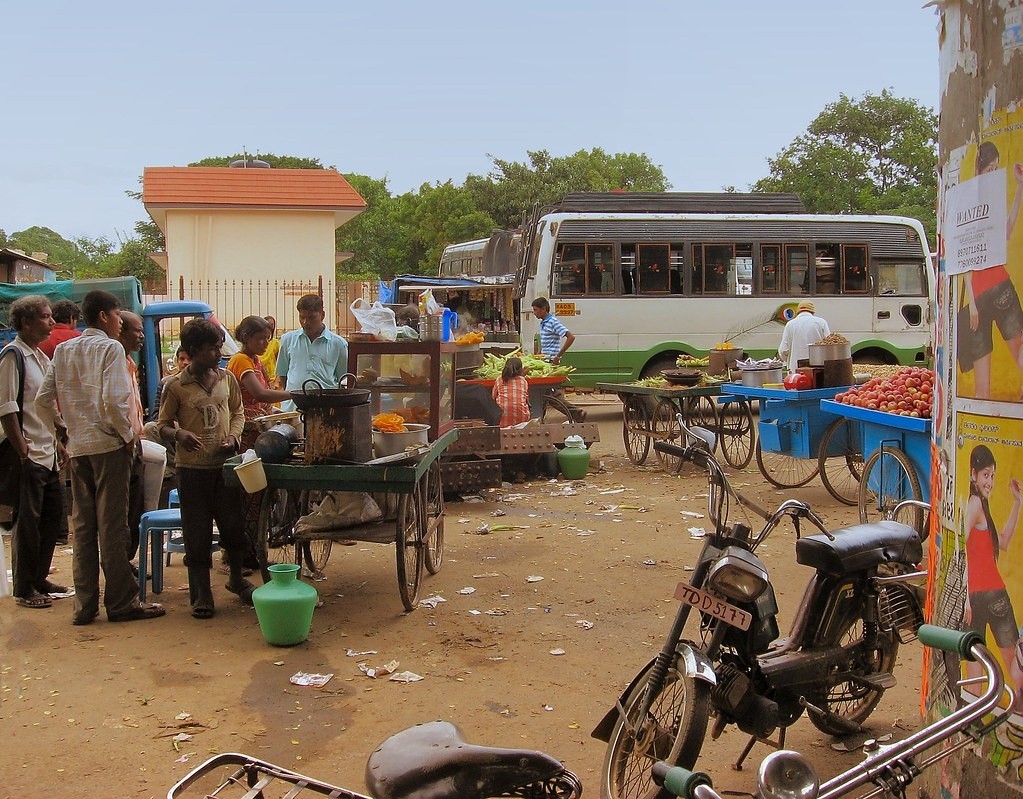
[192,603,214,619]
[225,579,257,603]
[217,563,253,576]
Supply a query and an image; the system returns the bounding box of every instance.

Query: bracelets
[174,428,181,442]
[555,354,560,359]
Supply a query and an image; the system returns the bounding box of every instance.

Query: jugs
[437,307,457,341]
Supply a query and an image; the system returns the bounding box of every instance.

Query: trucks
[0,275,243,422]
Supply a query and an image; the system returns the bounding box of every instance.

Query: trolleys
[222,429,458,611]
[716,383,932,542]
[597,380,755,475]
[454,376,573,424]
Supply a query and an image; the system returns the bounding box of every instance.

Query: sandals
[576,409,586,423]
[40,581,68,593]
[13,590,52,607]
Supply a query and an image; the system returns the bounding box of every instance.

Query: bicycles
[168,621,1015,799]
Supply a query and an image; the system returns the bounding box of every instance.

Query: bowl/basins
[664,376,700,388]
[807,343,852,366]
[709,349,743,376]
[741,369,782,388]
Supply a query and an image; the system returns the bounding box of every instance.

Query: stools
[165,489,221,567]
[138,508,182,602]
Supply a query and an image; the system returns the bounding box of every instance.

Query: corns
[456,331,485,345]
[551,366,573,375]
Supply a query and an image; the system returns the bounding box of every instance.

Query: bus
[513,195,936,416]
[438,230,524,323]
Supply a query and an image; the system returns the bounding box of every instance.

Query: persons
[34,288,165,625]
[254,316,283,408]
[963,142,1023,400]
[526,296,587,424]
[157,318,258,619]
[143,346,192,478]
[116,311,153,580]
[396,303,419,336]
[0,294,68,608]
[778,300,830,375]
[269,294,360,548]
[801,251,840,293]
[956,444,1022,697]
[492,357,530,427]
[36,300,82,545]
[226,315,292,451]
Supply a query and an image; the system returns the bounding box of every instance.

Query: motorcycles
[591,410,931,799]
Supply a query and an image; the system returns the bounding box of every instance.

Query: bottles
[470,319,516,334]
[252,564,318,646]
[557,439,590,480]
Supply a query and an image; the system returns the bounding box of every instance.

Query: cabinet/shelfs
[347,340,458,444]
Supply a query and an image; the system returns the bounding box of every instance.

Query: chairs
[665,269,681,294]
[600,271,633,294]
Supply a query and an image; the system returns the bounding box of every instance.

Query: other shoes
[129,562,152,579]
[108,602,165,620]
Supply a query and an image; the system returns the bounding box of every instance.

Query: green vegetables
[633,359,727,386]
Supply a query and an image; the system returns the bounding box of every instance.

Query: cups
[233,458,268,493]
[419,315,443,343]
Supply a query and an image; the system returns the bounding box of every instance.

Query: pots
[372,424,431,456]
[289,373,371,409]
[253,410,302,447]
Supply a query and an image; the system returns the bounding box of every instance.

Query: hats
[795,300,814,315]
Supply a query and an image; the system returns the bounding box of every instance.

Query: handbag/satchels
[0,438,21,531]
[350,296,397,341]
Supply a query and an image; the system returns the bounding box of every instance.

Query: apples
[836,365,934,418]
